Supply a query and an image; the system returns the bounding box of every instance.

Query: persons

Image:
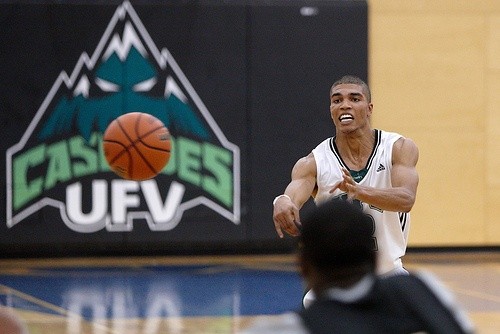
[235,197,476,334]
[272,75,419,311]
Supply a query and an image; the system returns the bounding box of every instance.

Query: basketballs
[102,113,174,181]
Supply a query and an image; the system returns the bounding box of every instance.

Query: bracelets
[273,193,291,205]
